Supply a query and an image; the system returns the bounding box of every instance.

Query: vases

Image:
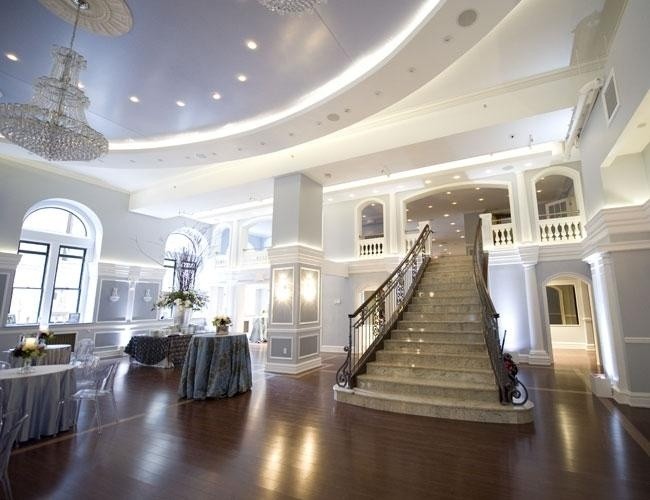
[38,341,47,346]
[20,358,34,373]
[216,325,229,335]
[176,306,191,329]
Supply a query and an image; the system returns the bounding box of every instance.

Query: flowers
[32,330,58,340]
[151,286,209,314]
[212,316,233,329]
[4,341,46,367]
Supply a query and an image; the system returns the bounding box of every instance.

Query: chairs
[70,338,96,376]
[1,413,29,499]
[71,348,125,436]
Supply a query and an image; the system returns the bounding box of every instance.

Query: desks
[0,363,80,448]
[9,343,72,367]
[128,326,207,368]
[178,332,252,399]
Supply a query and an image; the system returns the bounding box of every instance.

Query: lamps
[142,289,153,303]
[127,272,139,290]
[109,287,119,303]
[0,2,135,163]
